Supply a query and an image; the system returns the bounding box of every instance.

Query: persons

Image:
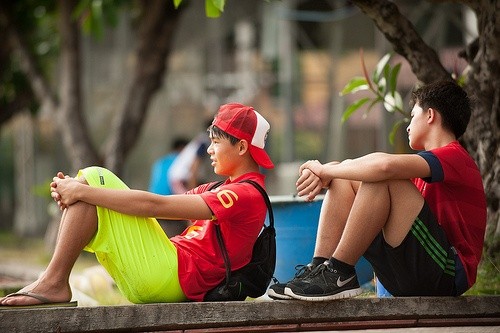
[267,80,487,303]
[149,118,214,195]
[0,100,275,315]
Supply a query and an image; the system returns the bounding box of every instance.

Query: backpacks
[202,179,276,303]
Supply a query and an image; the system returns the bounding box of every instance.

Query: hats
[211,103,274,169]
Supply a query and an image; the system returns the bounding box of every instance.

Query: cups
[376,277,392,299]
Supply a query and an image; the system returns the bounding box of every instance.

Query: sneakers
[267,264,319,300]
[284,263,362,301]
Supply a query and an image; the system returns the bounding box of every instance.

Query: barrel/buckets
[268,196,374,287]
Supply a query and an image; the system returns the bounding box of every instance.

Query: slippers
[0,293,79,310]
